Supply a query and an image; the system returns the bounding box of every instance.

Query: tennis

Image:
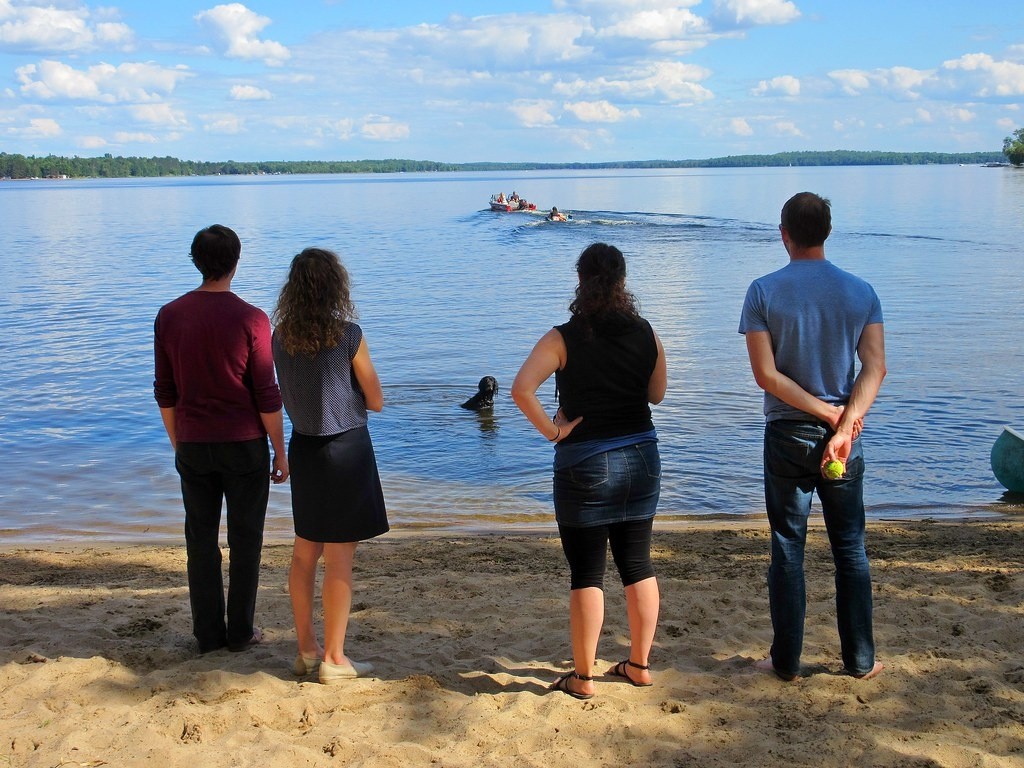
[823,459,844,479]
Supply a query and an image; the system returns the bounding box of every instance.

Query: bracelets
[550,428,560,441]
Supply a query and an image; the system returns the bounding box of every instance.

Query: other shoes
[319,657,376,685]
[293,653,323,676]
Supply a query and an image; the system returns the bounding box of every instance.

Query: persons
[511,243,667,699]
[738,192,886,681]
[490,191,533,211]
[154,224,289,650]
[548,206,559,218]
[271,247,390,683]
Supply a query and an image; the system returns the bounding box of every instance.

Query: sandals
[607,659,653,687]
[548,669,593,700]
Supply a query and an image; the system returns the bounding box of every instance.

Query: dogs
[461,376,499,411]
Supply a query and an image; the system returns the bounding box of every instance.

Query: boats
[547,214,576,223]
[958,164,964,166]
[980,162,1010,167]
[489,193,538,213]
[991,425,1024,495]
